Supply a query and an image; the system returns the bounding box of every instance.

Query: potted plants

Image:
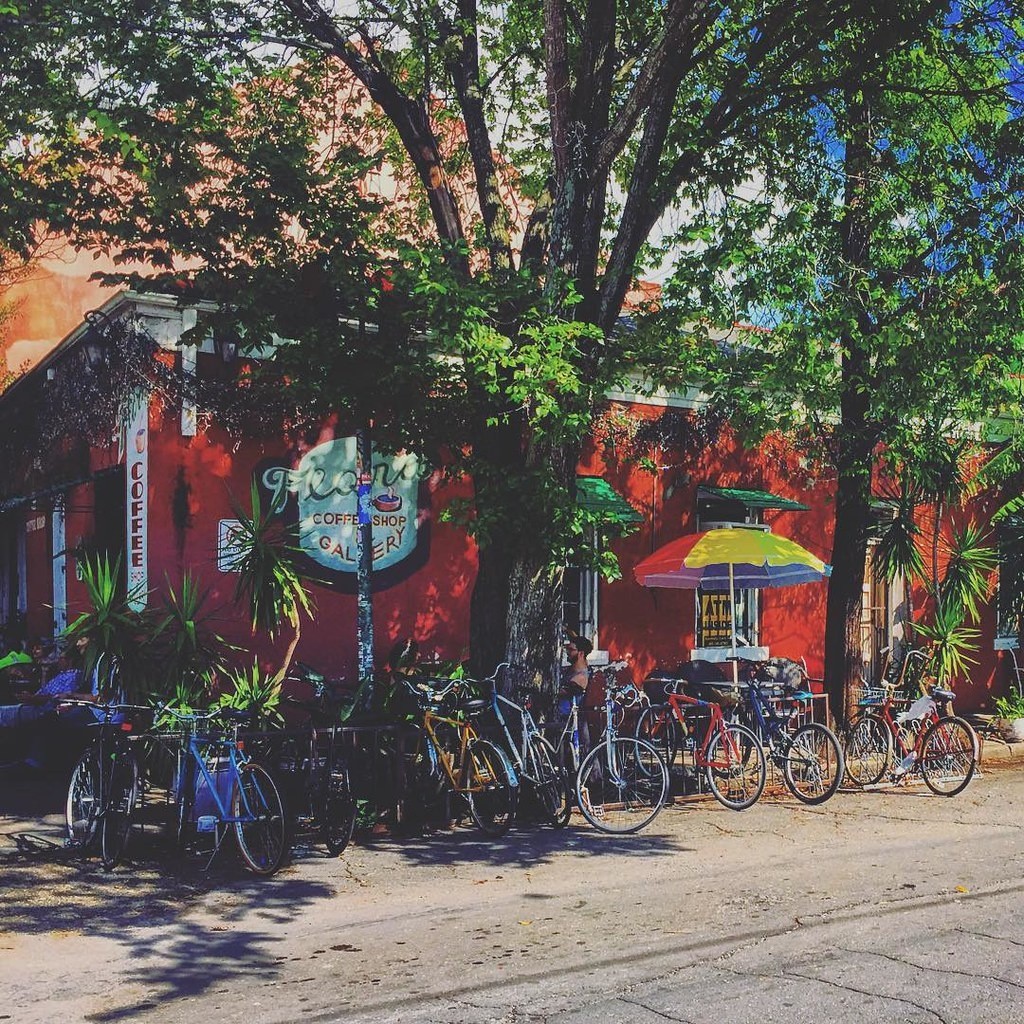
[991,686,1024,744]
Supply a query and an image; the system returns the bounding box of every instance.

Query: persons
[0,629,126,726]
[558,626,593,816]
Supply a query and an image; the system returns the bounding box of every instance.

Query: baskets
[850,686,904,707]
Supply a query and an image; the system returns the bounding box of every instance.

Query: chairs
[645,655,830,748]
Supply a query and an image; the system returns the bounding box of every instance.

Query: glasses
[567,647,579,653]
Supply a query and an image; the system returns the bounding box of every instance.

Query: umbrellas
[633,524,835,750]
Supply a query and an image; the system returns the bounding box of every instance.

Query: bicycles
[248,656,845,856]
[153,701,288,879]
[842,644,980,797]
[52,696,153,869]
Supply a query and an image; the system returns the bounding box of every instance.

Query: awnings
[698,485,812,512]
[575,476,645,521]
